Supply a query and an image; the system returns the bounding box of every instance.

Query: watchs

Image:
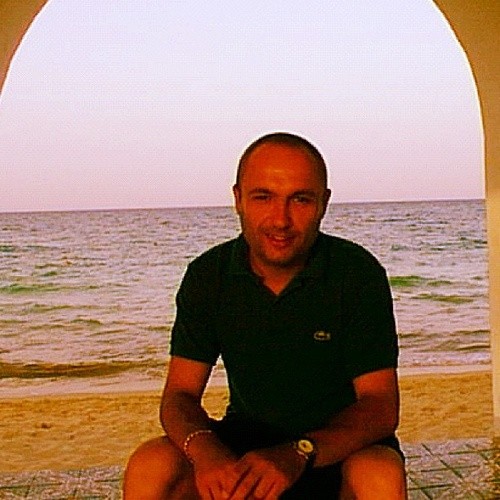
[287,433,319,475]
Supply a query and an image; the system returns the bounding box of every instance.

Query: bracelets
[183,429,216,464]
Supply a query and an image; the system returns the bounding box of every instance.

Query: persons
[123,132,409,500]
